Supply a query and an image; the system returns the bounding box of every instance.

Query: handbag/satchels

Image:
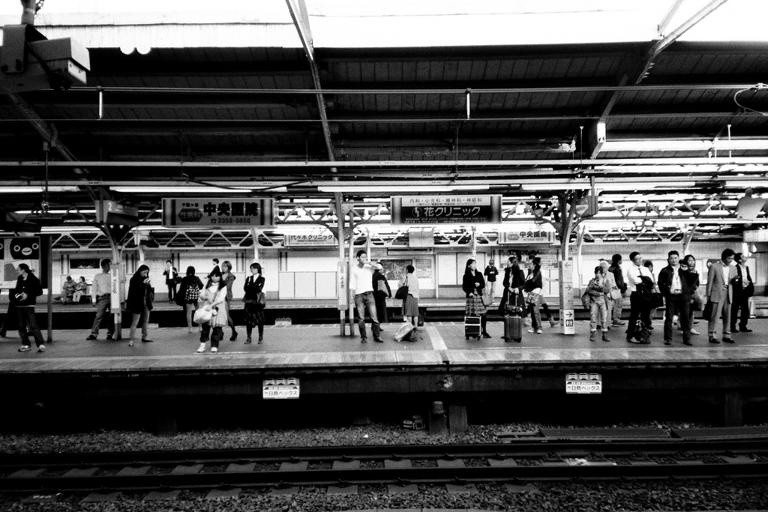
[581,294,590,310]
[703,297,713,322]
[245,293,265,308]
[193,305,211,324]
[396,286,408,299]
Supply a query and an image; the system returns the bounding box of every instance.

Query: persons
[348,249,384,344]
[462,252,560,338]
[11,263,47,352]
[87,259,116,342]
[583,246,755,344]
[372,262,392,331]
[72,276,86,305]
[53,276,77,302]
[125,265,154,347]
[163,256,267,354]
[401,265,419,329]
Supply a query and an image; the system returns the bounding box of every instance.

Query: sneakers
[18,344,45,352]
[196,342,217,352]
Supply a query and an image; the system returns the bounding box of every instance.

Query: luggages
[464,294,481,340]
[504,289,521,342]
